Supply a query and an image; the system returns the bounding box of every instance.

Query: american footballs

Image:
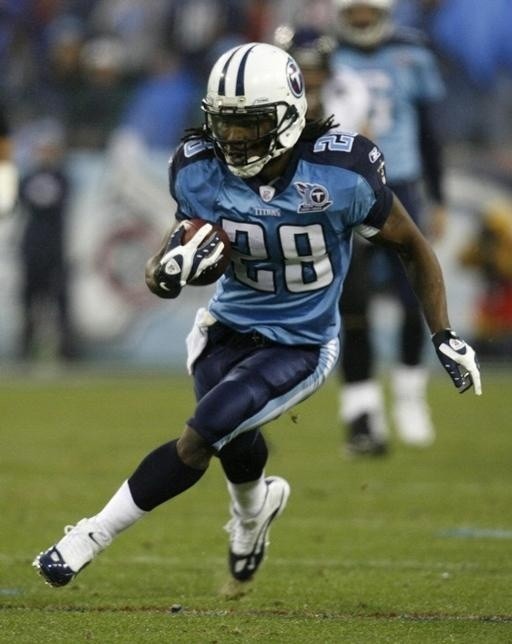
[175,218,231,286]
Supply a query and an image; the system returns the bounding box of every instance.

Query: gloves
[429,327,483,397]
[154,221,226,298]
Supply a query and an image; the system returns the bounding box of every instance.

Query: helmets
[199,43,311,177]
[332,0,394,49]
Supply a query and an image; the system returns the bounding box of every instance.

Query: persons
[36,43,482,589]
[285,1,447,456]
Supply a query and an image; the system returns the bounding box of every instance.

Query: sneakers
[340,363,435,456]
[34,516,109,588]
[227,472,289,583]
